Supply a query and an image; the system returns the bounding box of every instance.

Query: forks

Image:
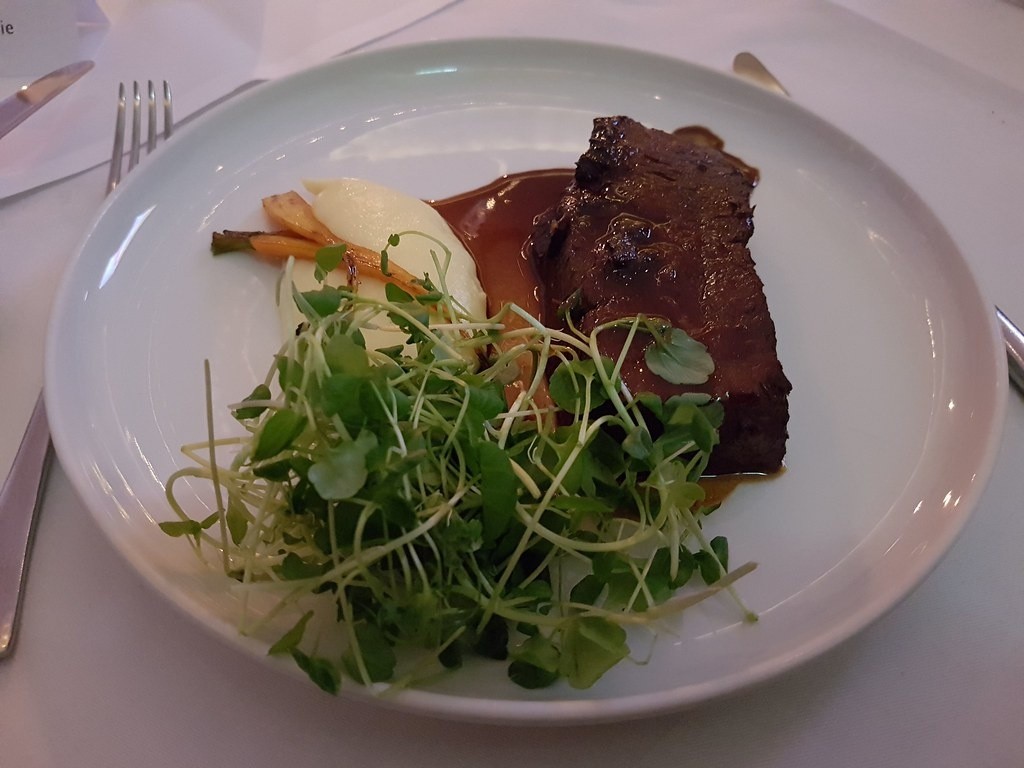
[0,80,173,658]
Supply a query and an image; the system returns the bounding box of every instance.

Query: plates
[43,36,1010,728]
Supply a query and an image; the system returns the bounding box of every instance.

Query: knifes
[732,51,1024,394]
[0,61,96,138]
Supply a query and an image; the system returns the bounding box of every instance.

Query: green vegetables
[159,228,760,702]
